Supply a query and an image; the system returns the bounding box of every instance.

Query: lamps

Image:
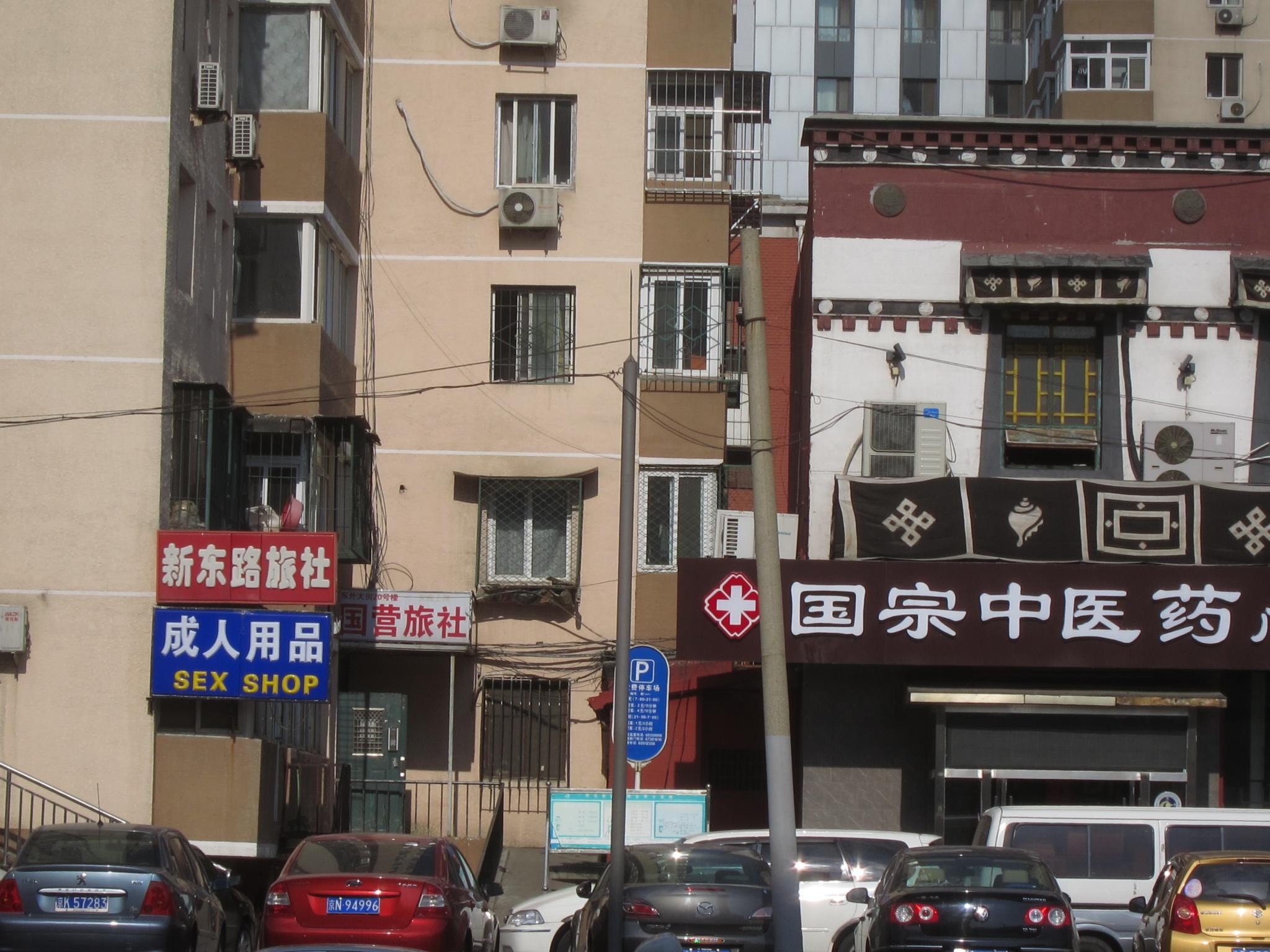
[1178,355,1195,386]
[886,343,906,379]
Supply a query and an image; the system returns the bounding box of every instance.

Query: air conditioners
[499,188,558,227]
[714,509,799,559]
[232,114,257,157]
[1216,8,1242,24]
[0,605,29,653]
[498,5,556,46]
[1221,99,1245,118]
[197,62,226,110]
[1142,421,1235,482]
[862,401,947,477]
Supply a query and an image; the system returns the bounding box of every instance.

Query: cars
[191,844,258,952]
[1128,850,1270,952]
[846,845,1080,952]
[0,820,231,952]
[258,832,504,952]
[571,843,773,952]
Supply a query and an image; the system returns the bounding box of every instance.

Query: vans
[965,806,1270,952]
[499,828,943,952]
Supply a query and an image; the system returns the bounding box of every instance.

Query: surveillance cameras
[1179,353,1193,373]
[894,344,907,361]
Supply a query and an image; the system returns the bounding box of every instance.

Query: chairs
[917,868,947,882]
[1002,867,1030,884]
